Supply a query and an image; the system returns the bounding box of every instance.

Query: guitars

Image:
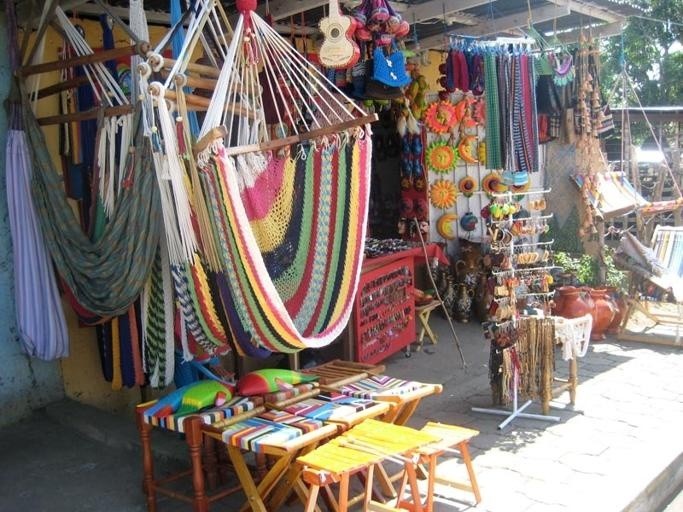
[319,0,360,72]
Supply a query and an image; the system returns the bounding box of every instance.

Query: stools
[202,404,340,512]
[301,357,386,394]
[295,437,386,512]
[134,393,267,512]
[342,416,443,512]
[324,372,443,498]
[218,379,320,485]
[413,300,443,346]
[265,387,397,511]
[396,422,480,512]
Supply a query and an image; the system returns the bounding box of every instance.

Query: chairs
[617,225,682,346]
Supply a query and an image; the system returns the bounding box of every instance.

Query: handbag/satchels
[326,42,412,100]
[536,48,616,144]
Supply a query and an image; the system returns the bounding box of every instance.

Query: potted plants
[552,251,614,341]
